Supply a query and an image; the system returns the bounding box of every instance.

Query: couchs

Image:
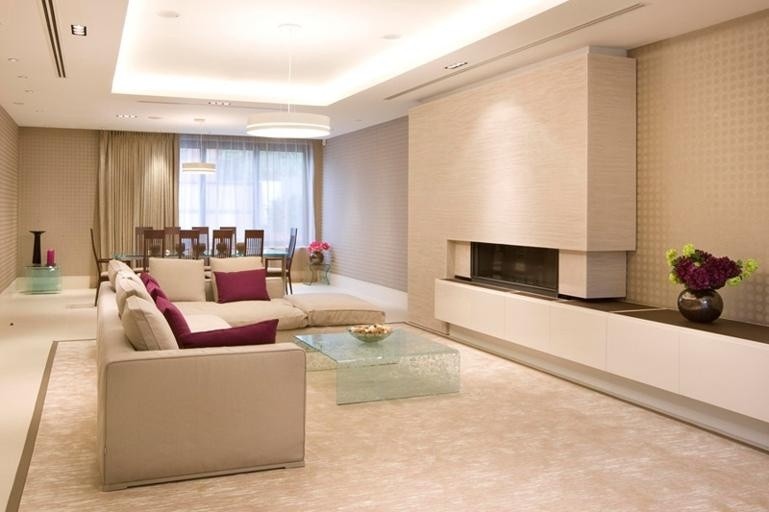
[96,276,387,494]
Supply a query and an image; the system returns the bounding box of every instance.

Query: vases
[26,228,46,264]
[676,286,724,324]
[308,251,324,264]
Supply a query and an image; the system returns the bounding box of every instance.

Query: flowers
[664,241,761,292]
[306,239,332,254]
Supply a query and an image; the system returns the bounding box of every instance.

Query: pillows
[107,256,279,350]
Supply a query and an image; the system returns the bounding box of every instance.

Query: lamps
[245,41,331,138]
[182,119,217,172]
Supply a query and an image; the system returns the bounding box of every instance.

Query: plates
[345,323,394,343]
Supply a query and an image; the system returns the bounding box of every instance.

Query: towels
[4,321,769,512]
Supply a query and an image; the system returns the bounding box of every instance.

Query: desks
[302,263,333,287]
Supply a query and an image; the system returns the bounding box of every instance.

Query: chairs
[90,227,298,307]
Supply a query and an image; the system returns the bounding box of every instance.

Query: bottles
[47,248,54,267]
